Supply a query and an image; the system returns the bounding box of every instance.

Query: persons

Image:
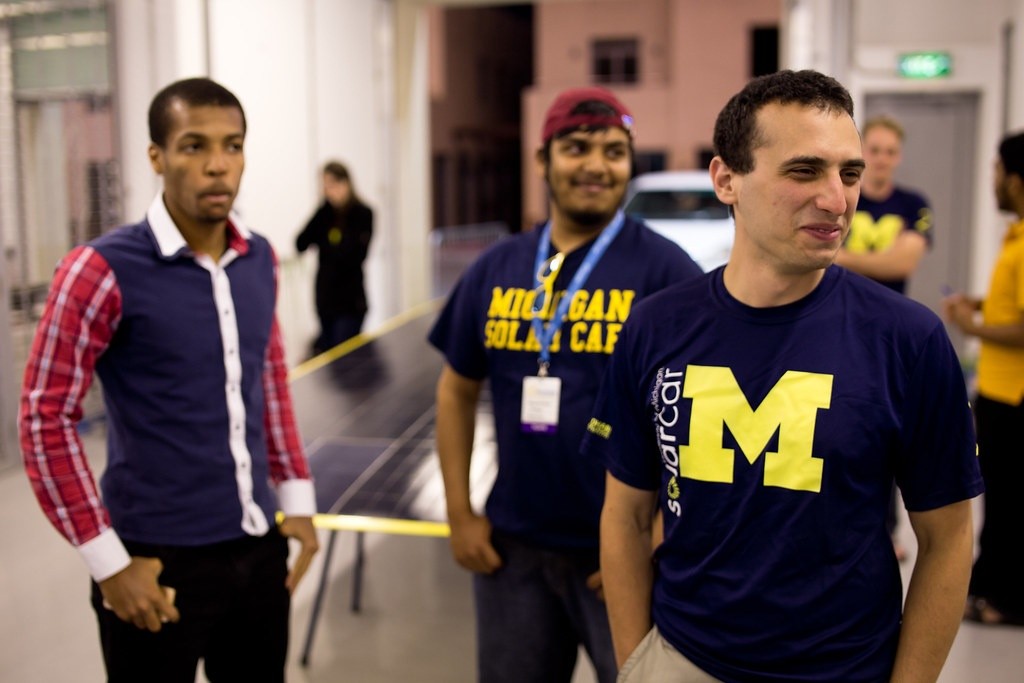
[599,67,986,683]
[17,77,319,683]
[943,127,1024,631]
[818,110,935,296]
[425,89,705,683]
[291,159,375,360]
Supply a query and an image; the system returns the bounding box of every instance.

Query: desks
[252,293,511,672]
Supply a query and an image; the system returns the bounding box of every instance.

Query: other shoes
[964,598,1012,624]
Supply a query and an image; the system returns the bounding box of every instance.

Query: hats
[543,88,632,141]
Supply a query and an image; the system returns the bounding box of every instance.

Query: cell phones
[102,586,176,622]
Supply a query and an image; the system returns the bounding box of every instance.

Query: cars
[621,168,735,275]
